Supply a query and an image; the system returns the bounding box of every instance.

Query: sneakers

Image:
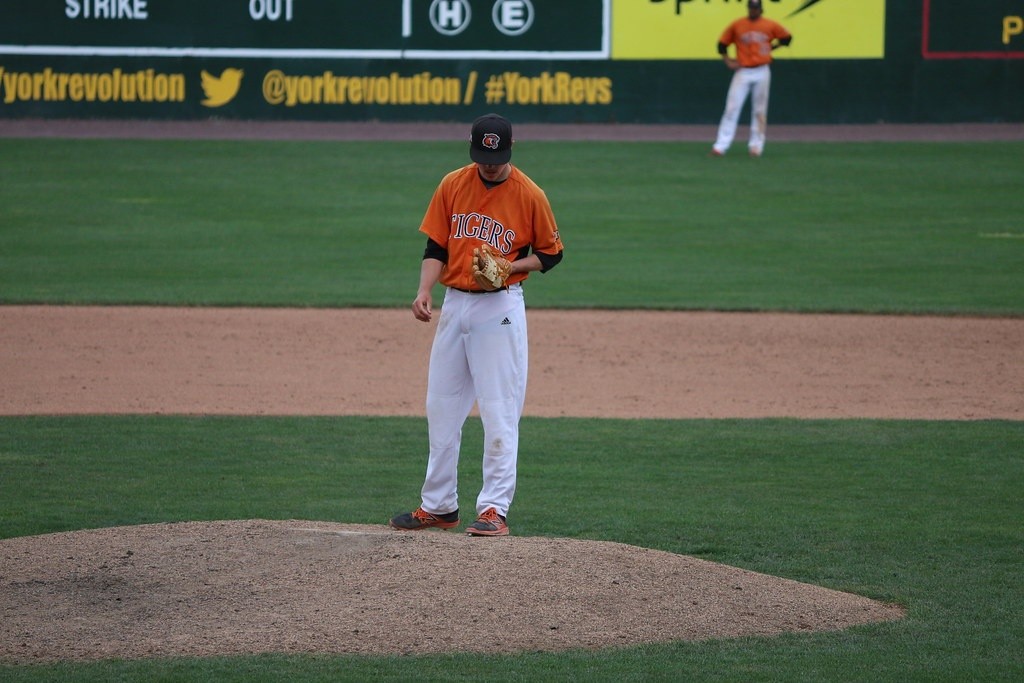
[389,506,460,530]
[465,507,510,535]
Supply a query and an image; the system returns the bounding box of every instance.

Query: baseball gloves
[470,243,514,294]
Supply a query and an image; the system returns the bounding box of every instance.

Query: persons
[711,0,793,156]
[390,113,564,536]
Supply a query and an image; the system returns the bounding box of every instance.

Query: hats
[749,0,760,8]
[470,114,512,164]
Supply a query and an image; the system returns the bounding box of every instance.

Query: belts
[452,281,522,293]
